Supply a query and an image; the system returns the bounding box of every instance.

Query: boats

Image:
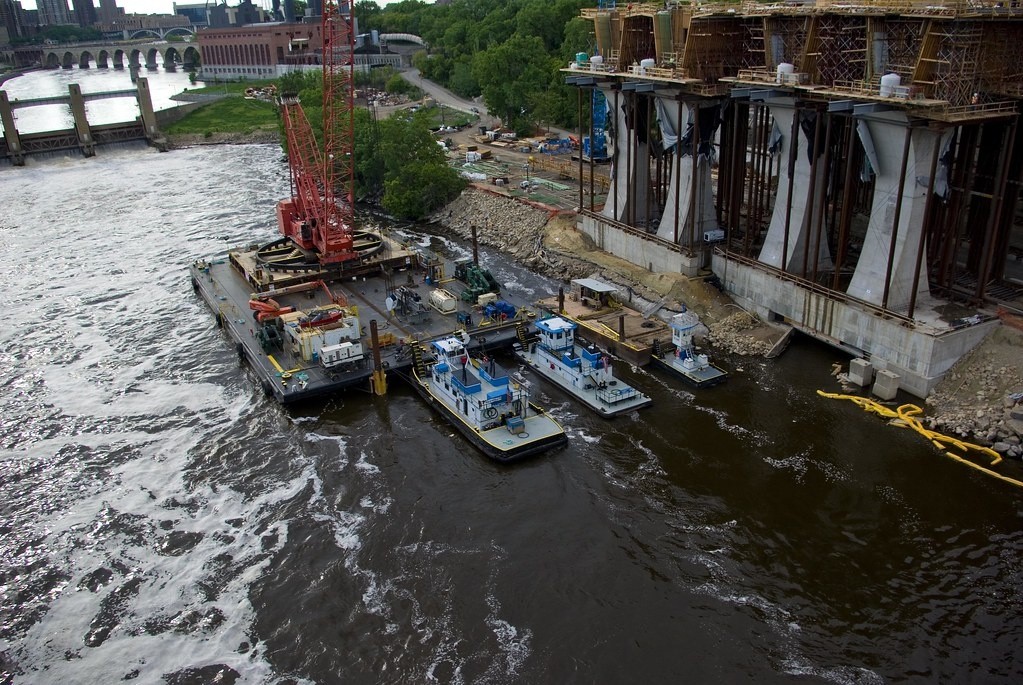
[533,279,729,387]
[512,315,652,417]
[409,337,568,461]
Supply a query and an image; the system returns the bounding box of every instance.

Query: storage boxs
[507,416,525,435]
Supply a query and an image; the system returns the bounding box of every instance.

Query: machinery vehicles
[248,281,348,326]
